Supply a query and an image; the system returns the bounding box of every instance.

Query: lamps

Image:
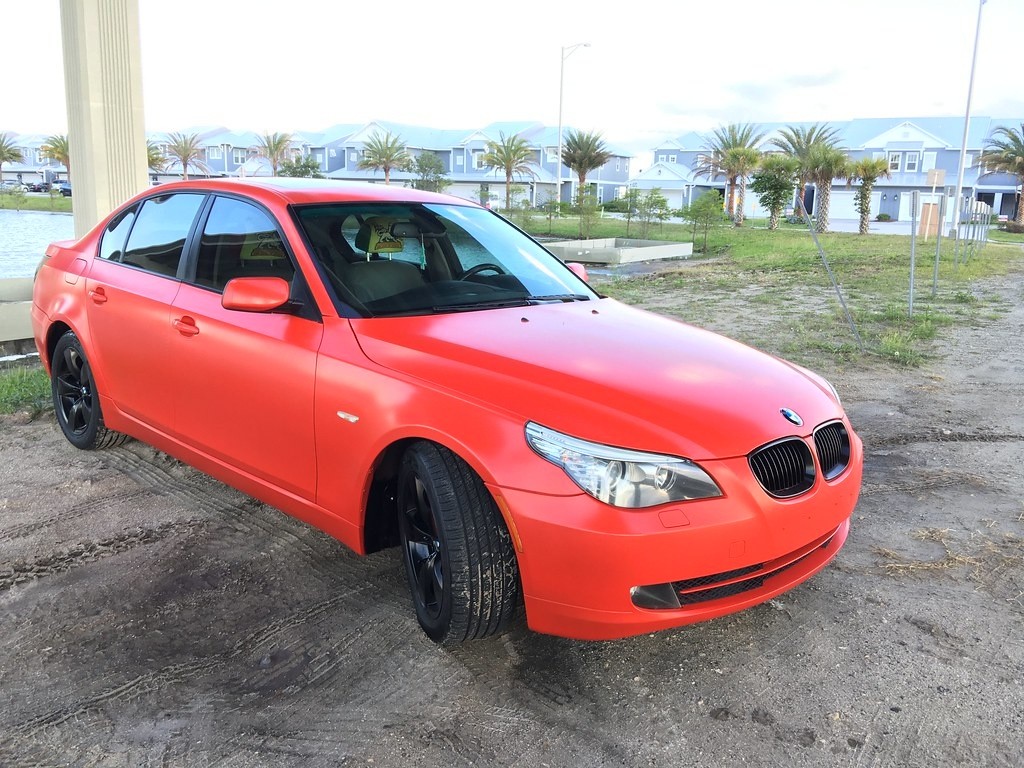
[966,196,969,202]
[883,194,887,199]
[894,194,898,200]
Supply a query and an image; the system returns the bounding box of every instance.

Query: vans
[52,179,70,190]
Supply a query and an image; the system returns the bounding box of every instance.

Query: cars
[29,176,865,647]
[60,184,72,196]
[29,182,51,193]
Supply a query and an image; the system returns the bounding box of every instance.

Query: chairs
[233,222,293,283]
[349,217,426,301]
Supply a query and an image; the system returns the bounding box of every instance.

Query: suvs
[0,179,29,193]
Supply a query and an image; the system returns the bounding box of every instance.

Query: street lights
[557,42,591,214]
